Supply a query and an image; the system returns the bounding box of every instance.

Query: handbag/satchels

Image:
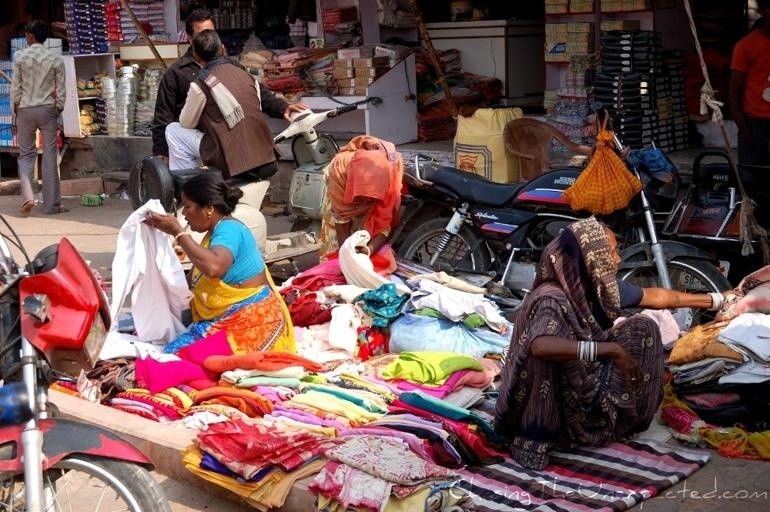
[560,110,642,215]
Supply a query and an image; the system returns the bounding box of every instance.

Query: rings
[631,376,637,381]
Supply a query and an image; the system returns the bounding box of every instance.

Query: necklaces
[214,215,226,230]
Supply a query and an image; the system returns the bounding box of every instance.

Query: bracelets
[576,340,598,363]
[706,292,724,312]
[175,232,191,245]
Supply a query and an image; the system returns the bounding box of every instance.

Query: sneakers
[57,205,70,212]
[19,200,34,213]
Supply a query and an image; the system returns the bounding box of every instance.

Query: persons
[6,23,27,60]
[726,0,769,166]
[316,134,406,281]
[490,216,746,448]
[163,27,284,180]
[140,175,298,363]
[9,20,70,215]
[149,11,308,164]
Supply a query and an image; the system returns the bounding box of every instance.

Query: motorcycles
[127,104,358,290]
[1,212,175,512]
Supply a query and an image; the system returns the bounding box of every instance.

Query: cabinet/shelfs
[60,1,189,147]
[542,1,689,155]
